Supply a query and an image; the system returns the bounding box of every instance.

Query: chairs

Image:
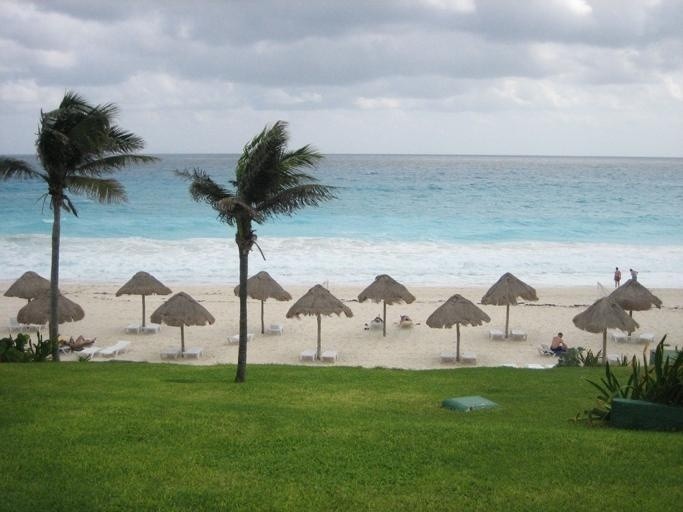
[8,315,48,336]
[300,346,337,361]
[439,329,527,365]
[226,320,286,346]
[637,332,656,343]
[126,318,164,335]
[608,332,626,342]
[58,333,133,363]
[160,342,204,361]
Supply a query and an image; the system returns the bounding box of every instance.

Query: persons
[628,269,638,281]
[548,332,568,356]
[612,267,621,286]
